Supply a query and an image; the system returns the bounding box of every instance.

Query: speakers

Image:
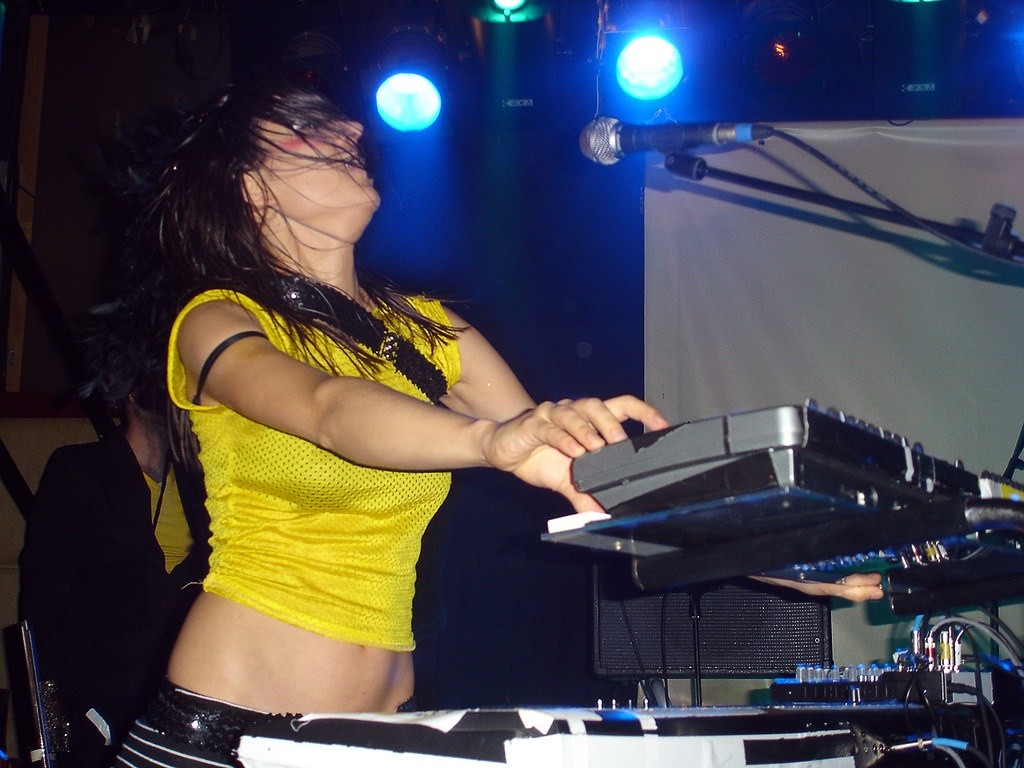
[6,620,75,768]
[592,551,835,682]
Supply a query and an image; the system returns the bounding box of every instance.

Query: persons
[109,77,670,768]
[19,377,213,768]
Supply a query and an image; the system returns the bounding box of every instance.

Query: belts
[156,687,302,753]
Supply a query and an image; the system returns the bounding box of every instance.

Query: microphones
[579,116,775,167]
